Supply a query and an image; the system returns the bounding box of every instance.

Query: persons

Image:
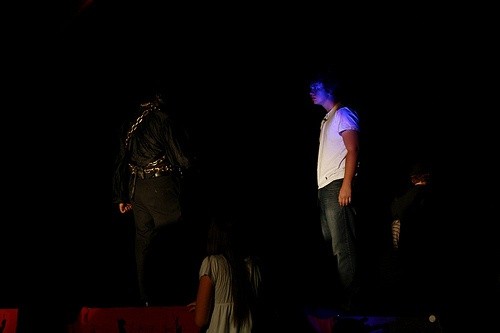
[194,219,264,333]
[392,160,448,323]
[309,69,368,318]
[111,91,192,307]
